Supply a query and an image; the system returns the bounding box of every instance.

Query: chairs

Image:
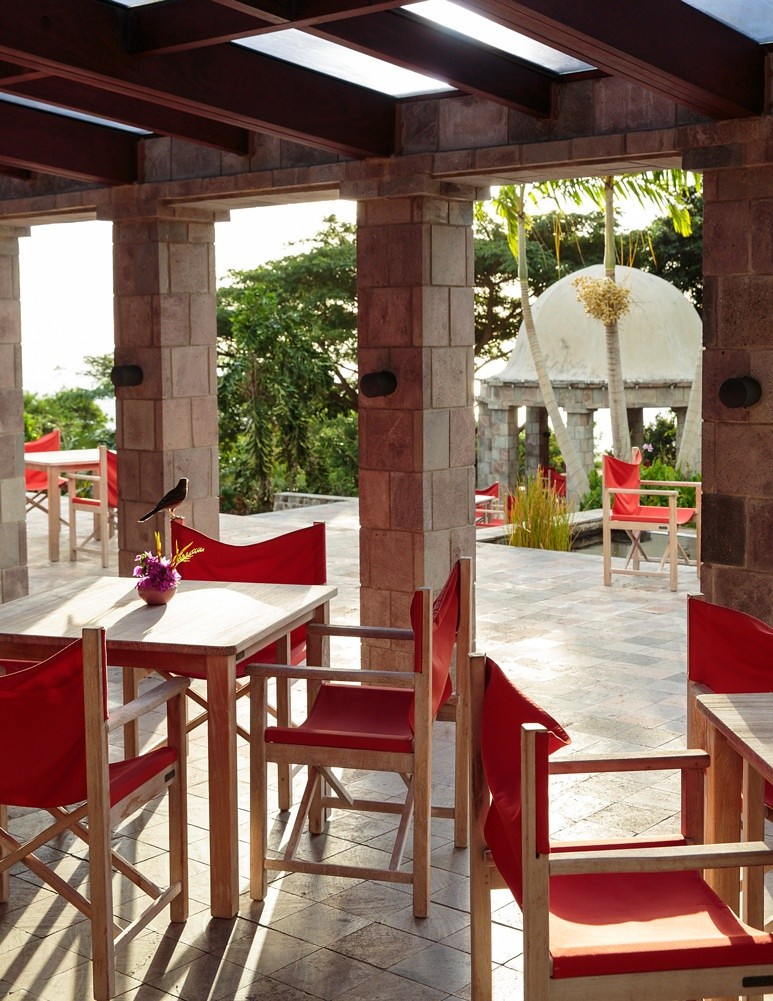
[0,428,773,1001]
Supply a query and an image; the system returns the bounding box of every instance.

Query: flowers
[132,531,205,591]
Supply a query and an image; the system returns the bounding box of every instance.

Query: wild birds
[137,477,189,522]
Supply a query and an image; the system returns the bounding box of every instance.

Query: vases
[139,585,177,606]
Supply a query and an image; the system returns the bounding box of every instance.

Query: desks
[694,688,773,1000]
[0,574,340,917]
[24,449,100,562]
[475,495,496,525]
